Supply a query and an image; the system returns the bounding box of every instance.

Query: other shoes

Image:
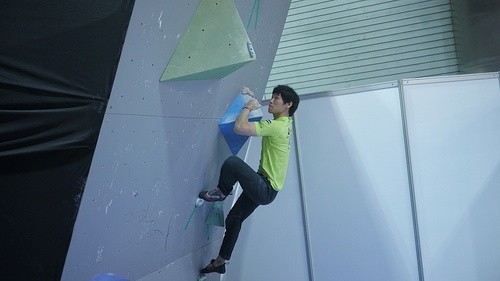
[198,189,227,202]
[200,259,229,274]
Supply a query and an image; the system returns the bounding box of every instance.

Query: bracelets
[242,107,252,112]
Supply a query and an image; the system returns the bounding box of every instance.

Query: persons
[198,84,300,274]
